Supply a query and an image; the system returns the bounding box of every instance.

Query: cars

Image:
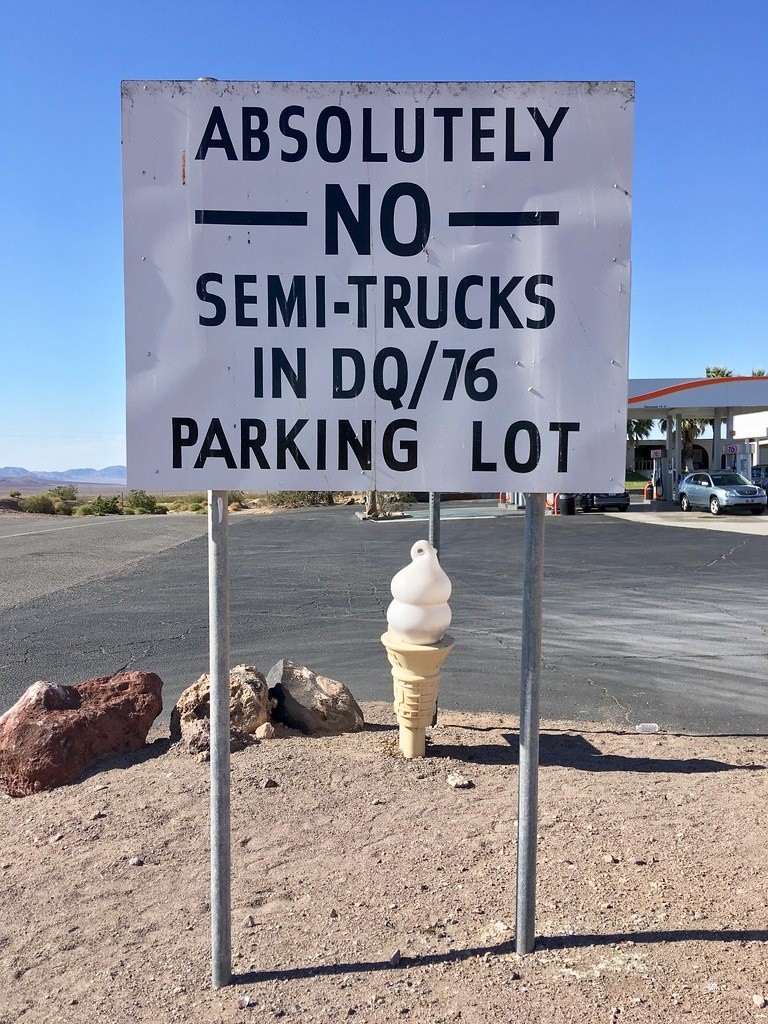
[676,469,767,516]
[751,464,768,494]
[572,489,630,512]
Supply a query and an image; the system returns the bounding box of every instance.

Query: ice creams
[380,539,456,760]
